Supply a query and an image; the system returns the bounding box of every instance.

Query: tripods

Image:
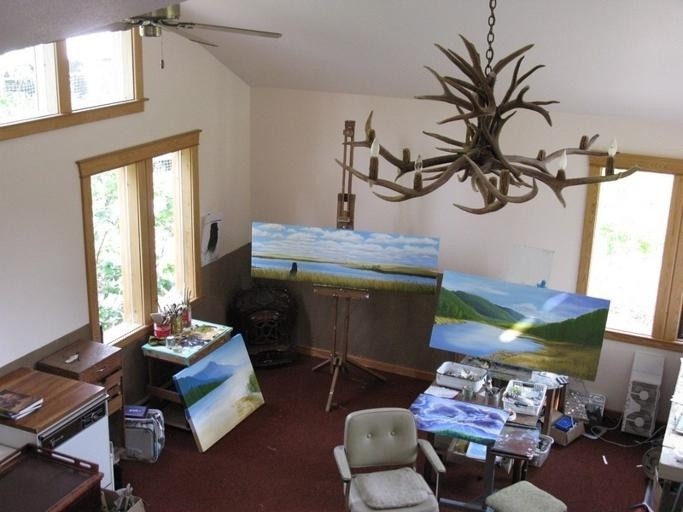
[311,297,386,412]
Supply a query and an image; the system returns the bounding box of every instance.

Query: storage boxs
[539,412,585,447]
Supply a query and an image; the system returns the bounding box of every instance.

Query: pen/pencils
[483,382,504,395]
[168,304,184,317]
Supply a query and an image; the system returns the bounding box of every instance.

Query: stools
[484,481,568,512]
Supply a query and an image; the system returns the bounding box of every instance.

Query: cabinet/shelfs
[37,340,125,456]
[0,443,105,512]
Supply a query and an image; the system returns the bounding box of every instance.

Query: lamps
[335,0,640,215]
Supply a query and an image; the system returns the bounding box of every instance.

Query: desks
[407,378,541,512]
[142,319,234,432]
[657,359,683,512]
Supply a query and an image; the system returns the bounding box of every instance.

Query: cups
[166,336,175,349]
[485,386,501,408]
[172,346,182,352]
[462,385,474,400]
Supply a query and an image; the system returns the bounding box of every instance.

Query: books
[0,389,43,420]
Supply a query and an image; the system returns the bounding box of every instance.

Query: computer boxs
[620,352,664,438]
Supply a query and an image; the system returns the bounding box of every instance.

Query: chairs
[334,408,446,512]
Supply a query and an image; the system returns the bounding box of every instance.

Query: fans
[44,4,284,47]
